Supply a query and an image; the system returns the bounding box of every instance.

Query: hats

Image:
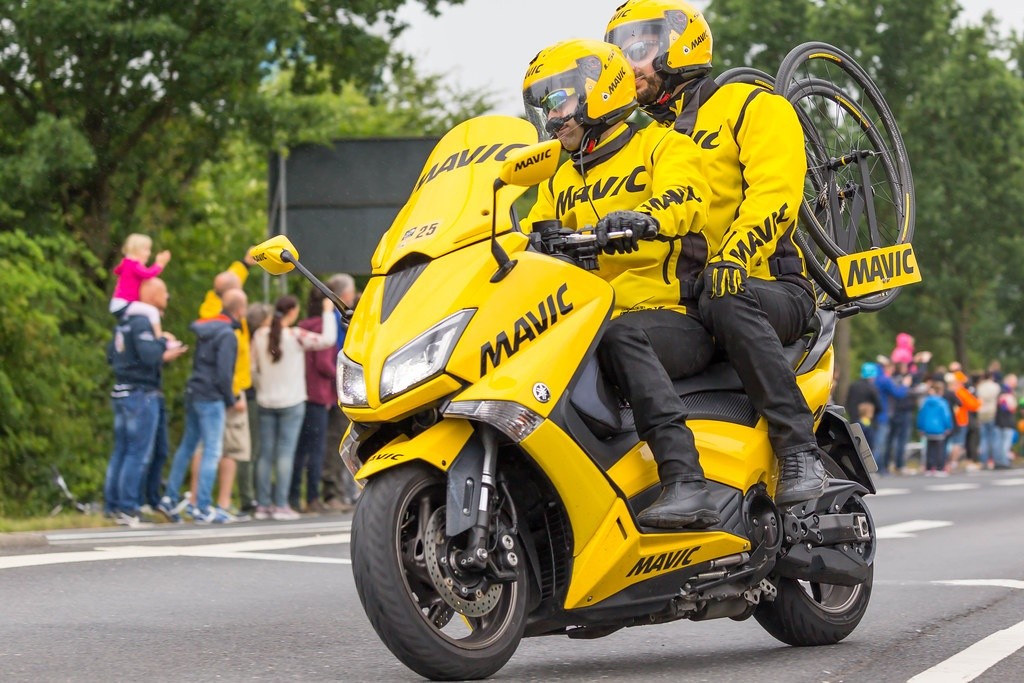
[860,361,876,380]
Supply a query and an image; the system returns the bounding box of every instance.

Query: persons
[514,38,725,532]
[103,245,364,524]
[108,234,176,344]
[605,0,830,506]
[846,333,1020,477]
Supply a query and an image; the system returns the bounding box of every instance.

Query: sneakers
[775,451,831,503]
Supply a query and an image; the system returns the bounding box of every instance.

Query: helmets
[605,1,713,74]
[523,39,637,126]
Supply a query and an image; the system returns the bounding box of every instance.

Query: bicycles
[713,39,917,320]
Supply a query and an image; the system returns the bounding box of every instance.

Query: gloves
[693,262,748,298]
[595,210,658,255]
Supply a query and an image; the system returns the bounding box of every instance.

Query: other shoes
[895,453,1024,478]
[105,495,352,529]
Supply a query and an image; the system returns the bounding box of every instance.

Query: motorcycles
[250,114,924,681]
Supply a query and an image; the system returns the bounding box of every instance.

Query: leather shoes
[635,480,722,528]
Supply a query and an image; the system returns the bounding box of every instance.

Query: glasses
[622,40,660,62]
[539,89,574,116]
